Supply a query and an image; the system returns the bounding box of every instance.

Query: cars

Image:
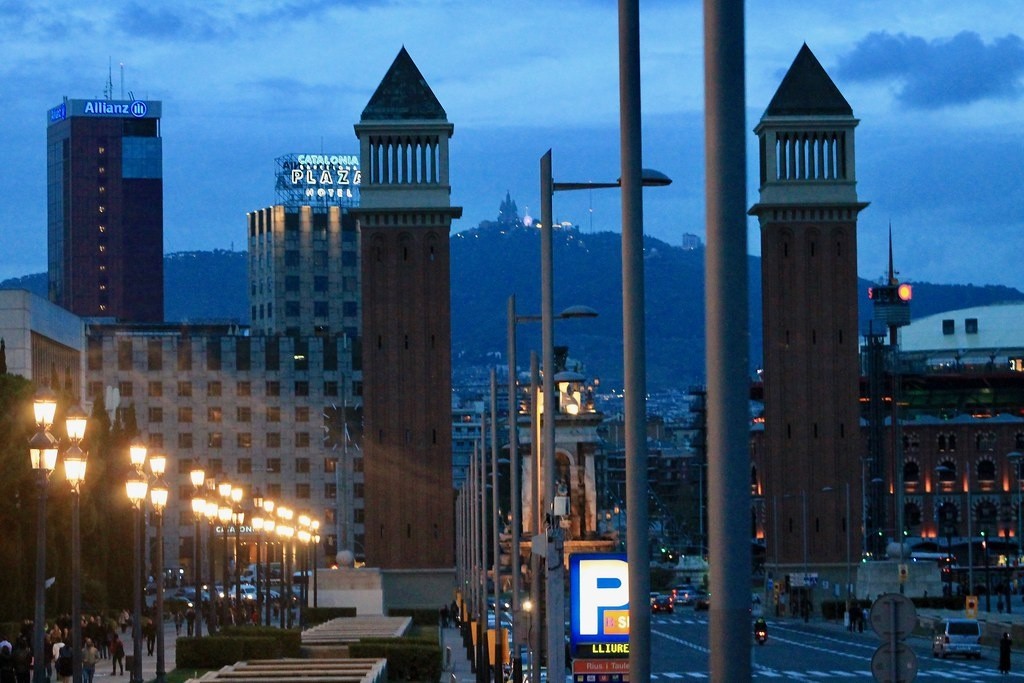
[648,584,710,615]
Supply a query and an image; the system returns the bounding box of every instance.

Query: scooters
[755,621,767,646]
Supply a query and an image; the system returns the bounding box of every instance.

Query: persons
[754,619,768,641]
[848,603,857,632]
[998,632,1012,676]
[791,595,798,618]
[754,616,767,630]
[450,600,459,627]
[440,604,449,627]
[800,595,813,623]
[0,575,301,682]
[857,608,864,633]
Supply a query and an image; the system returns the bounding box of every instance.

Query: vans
[931,618,982,660]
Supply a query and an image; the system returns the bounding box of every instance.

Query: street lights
[252,486,264,626]
[61,399,89,682]
[980,531,990,613]
[539,147,674,683]
[262,495,274,627]
[862,457,876,558]
[147,443,169,683]
[229,478,244,628]
[784,490,810,624]
[126,432,149,682]
[217,472,230,636]
[822,482,853,630]
[190,457,205,638]
[204,469,218,635]
[506,294,601,682]
[276,503,321,628]
[27,394,61,682]
[459,353,590,683]
